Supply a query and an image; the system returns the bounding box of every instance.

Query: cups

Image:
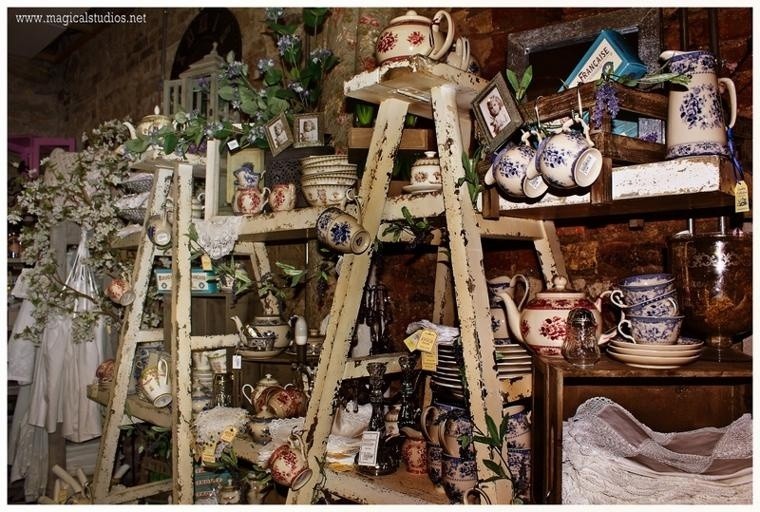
[246,334,276,353]
[610,272,685,345]
[103,271,136,306]
[265,433,314,492]
[314,197,371,255]
[484,116,604,201]
[398,401,531,504]
[146,203,174,247]
[94,345,227,414]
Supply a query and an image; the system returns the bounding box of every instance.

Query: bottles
[562,307,602,369]
[216,479,242,504]
[8,228,20,258]
[245,406,279,446]
[409,149,443,181]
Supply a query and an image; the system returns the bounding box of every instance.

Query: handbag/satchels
[561,397,753,504]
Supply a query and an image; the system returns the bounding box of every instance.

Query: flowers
[121,8,342,150]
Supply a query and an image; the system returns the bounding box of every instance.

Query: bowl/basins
[298,153,358,207]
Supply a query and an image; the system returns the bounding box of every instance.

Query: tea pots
[375,9,481,77]
[123,106,187,140]
[229,305,304,352]
[232,163,297,217]
[240,373,294,405]
[496,275,626,360]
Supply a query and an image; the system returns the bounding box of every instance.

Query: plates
[403,183,443,194]
[235,348,283,359]
[606,338,704,371]
[429,342,534,403]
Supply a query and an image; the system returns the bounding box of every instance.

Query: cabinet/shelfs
[7,54,575,505]
